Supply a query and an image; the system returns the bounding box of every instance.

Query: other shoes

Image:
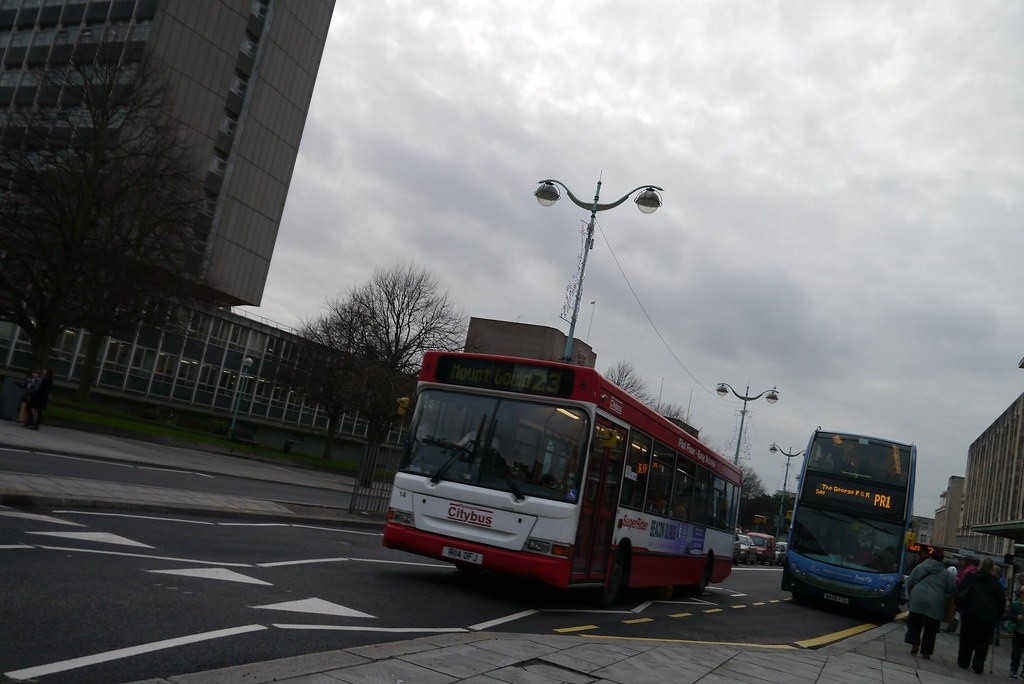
[1009,669,1019,678]
[922,651,932,659]
[909,644,920,655]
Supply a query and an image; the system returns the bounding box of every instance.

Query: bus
[380,350,746,606]
[780,424,917,616]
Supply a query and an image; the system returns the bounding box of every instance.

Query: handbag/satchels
[18,401,39,424]
[957,575,992,617]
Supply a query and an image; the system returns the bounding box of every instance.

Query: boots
[21,408,34,427]
[29,413,44,430]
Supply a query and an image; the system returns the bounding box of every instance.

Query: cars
[732,525,787,569]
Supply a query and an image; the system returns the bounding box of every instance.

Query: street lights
[770,442,808,539]
[533,167,664,363]
[715,380,780,468]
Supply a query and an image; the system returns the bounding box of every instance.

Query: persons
[942,557,1007,675]
[904,547,956,660]
[453,414,499,472]
[1011,585,1024,679]
[15,368,53,432]
